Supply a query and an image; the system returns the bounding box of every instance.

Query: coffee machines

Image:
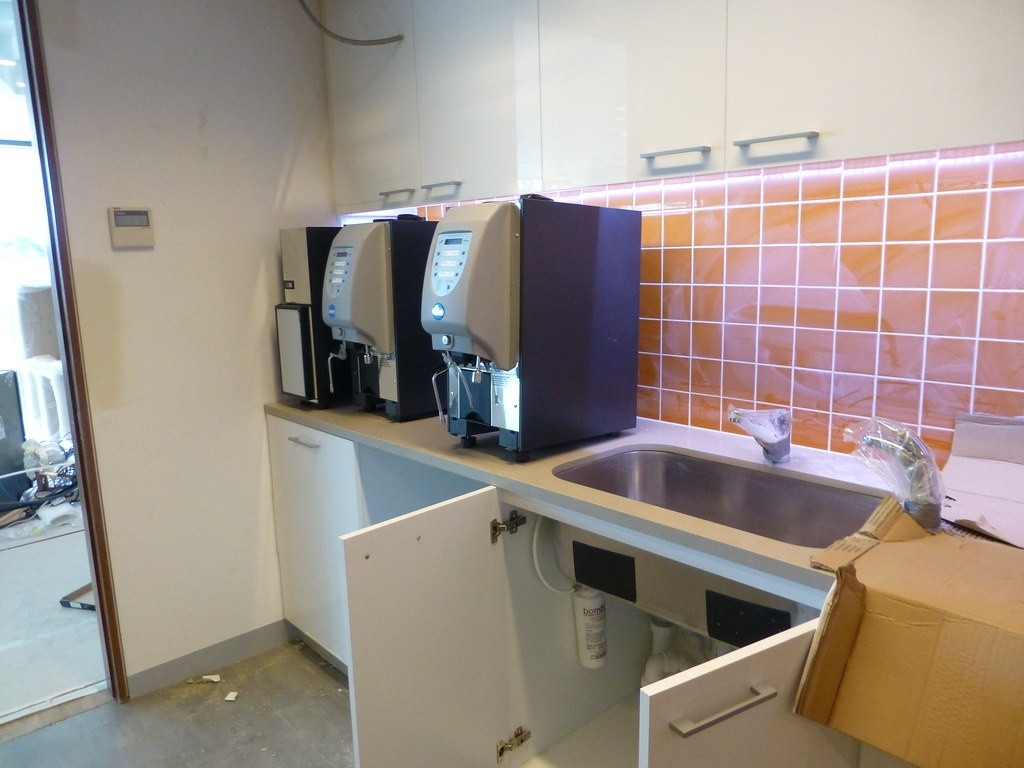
[420,195,642,461]
[317,215,447,417]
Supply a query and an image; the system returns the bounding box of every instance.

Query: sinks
[551,443,895,550]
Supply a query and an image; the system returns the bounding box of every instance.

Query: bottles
[572,590,607,669]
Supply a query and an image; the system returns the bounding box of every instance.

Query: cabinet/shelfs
[323,0,1024,217]
[267,415,359,666]
[338,486,859,768]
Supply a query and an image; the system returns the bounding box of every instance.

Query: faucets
[862,418,943,529]
[727,409,792,465]
